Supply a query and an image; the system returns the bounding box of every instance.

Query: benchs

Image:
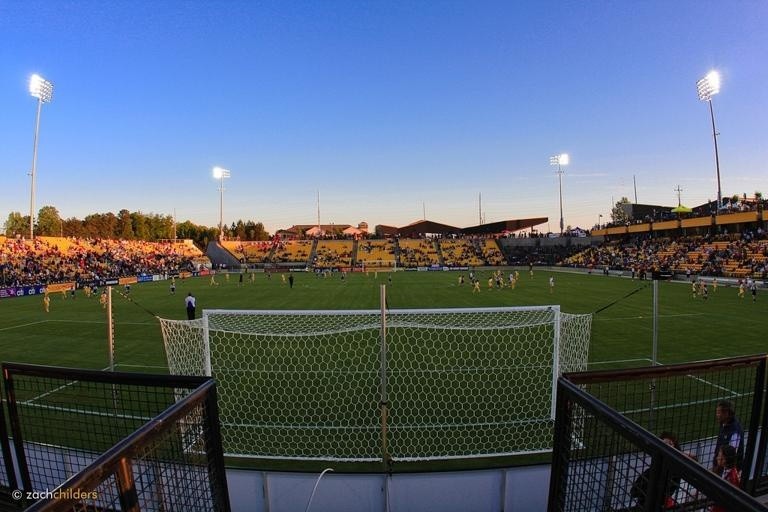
[654,241,768,279]
[220,240,505,268]
[0,236,208,273]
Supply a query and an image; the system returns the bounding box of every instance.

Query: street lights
[696,69,725,214]
[214,168,230,225]
[28,74,53,237]
[550,154,568,236]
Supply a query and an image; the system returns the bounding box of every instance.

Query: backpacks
[630,467,650,497]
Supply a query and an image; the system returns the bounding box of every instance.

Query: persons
[101,291,107,312]
[0,231,213,300]
[708,446,742,512]
[587,225,768,304]
[185,294,196,320]
[209,275,219,287]
[44,294,51,313]
[471,279,481,294]
[713,400,745,476]
[630,431,702,508]
[549,274,555,295]
[226,273,231,283]
